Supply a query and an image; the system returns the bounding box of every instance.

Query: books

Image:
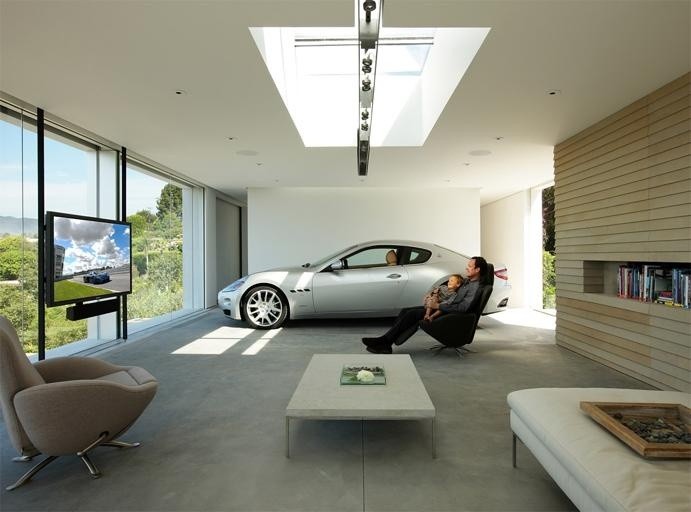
[618,264,691,311]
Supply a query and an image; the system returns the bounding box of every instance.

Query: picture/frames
[580,401,691,459]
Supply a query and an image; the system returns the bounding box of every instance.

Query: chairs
[0,316,157,491]
[419,264,494,359]
[386,251,398,266]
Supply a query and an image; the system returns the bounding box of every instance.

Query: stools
[507,387,691,512]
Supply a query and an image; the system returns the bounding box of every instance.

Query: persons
[423,275,464,323]
[362,257,488,354]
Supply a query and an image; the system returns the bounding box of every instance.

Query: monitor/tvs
[46,211,132,308]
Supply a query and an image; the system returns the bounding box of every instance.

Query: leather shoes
[362,338,392,354]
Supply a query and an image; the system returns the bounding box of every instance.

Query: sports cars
[217,239,511,329]
[83,271,109,284]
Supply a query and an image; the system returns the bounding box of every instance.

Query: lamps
[358,0,382,174]
